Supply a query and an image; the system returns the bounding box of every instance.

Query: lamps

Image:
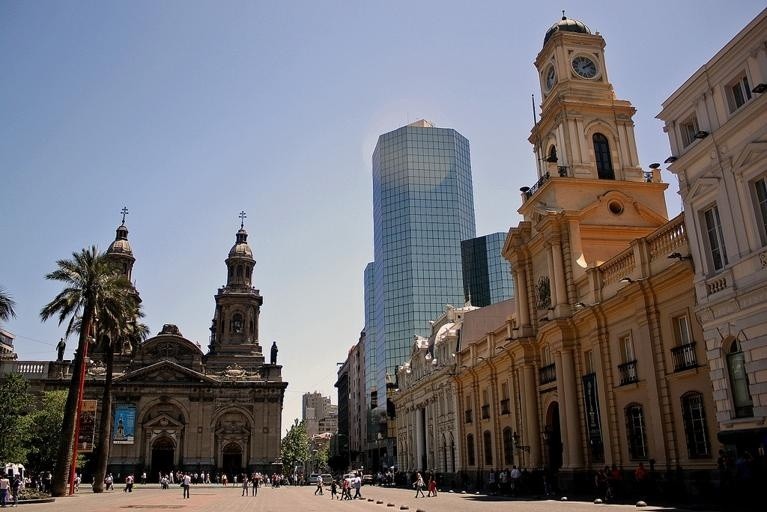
[752,83,767,94]
[619,277,634,284]
[574,303,586,308]
[664,157,678,163]
[511,432,523,452]
[541,424,552,440]
[667,252,692,262]
[694,131,708,139]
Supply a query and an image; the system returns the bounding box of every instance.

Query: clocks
[541,61,555,93]
[571,52,601,79]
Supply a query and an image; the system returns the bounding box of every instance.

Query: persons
[2,449,753,511]
[270,341,278,365]
[55,337,66,361]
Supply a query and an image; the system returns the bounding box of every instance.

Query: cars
[362,474,375,486]
[309,472,319,483]
[343,468,361,488]
[320,474,334,485]
